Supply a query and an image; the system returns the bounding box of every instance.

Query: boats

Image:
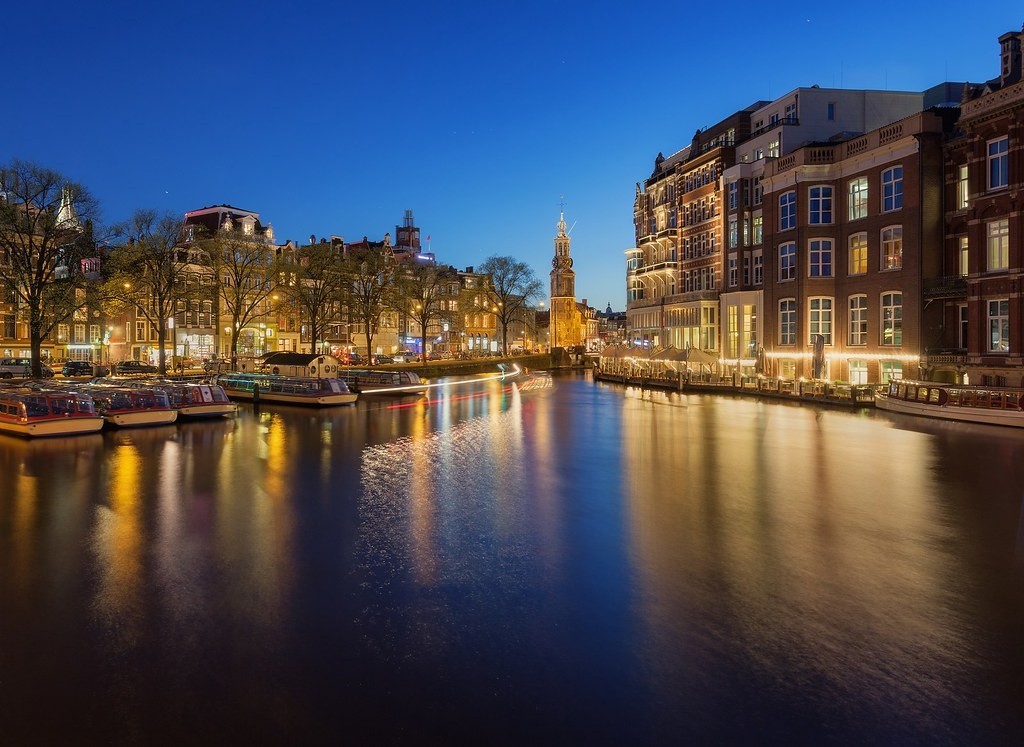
[11,381,178,427]
[0,384,106,440]
[91,380,239,419]
[339,370,430,397]
[198,372,359,407]
[873,378,1024,428]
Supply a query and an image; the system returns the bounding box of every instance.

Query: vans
[0,357,55,379]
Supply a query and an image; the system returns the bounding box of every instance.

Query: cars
[63,361,110,377]
[116,361,159,376]
[418,352,442,360]
[43,357,73,373]
[393,351,420,363]
[371,355,394,365]
[339,353,366,366]
